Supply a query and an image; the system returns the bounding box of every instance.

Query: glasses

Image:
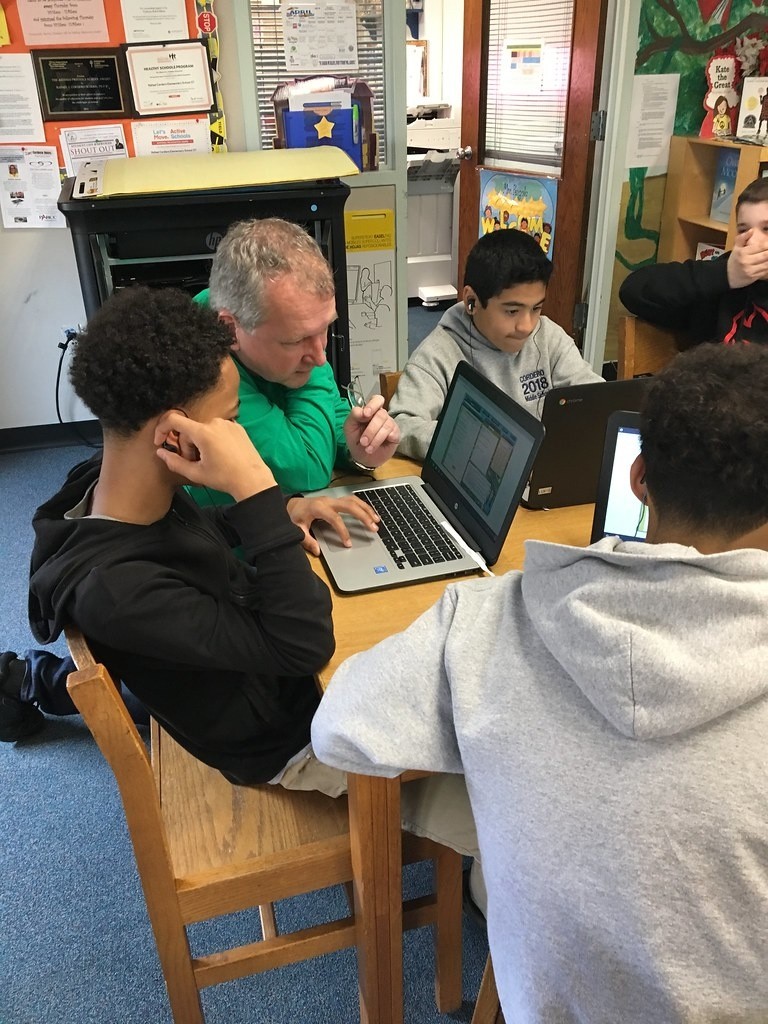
[341,375,367,410]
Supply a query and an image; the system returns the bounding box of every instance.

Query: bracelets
[352,459,376,472]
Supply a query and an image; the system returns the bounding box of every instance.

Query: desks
[303,455,599,1024]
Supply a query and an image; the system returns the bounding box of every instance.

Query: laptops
[300,361,546,595]
[517,377,657,510]
[588,409,649,545]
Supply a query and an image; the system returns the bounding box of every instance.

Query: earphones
[162,442,177,453]
[468,298,475,311]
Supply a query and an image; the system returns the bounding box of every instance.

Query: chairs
[65,622,465,1024]
[616,315,681,380]
[379,372,402,412]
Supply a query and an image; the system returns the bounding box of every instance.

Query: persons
[312,344,768,1024]
[9,165,18,176]
[618,176,768,351]
[390,230,609,460]
[28,285,494,915]
[115,139,123,149]
[182,217,401,510]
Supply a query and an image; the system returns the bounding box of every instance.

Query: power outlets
[60,324,83,354]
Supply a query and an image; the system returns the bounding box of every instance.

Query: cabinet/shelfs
[656,133,768,271]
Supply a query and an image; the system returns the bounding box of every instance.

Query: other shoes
[0,650,48,744]
